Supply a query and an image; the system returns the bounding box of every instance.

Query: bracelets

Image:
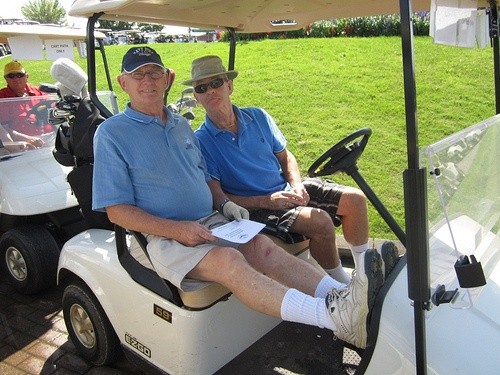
[220,199,230,215]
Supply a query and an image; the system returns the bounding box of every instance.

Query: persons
[0,61,58,147]
[93,47,400,349]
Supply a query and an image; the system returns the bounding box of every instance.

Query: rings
[285,202,288,208]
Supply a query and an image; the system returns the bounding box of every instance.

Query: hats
[4,60,26,76]
[121,46,167,74]
[180,55,239,87]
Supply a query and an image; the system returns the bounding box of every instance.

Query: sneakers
[326,249,382,349]
[376,241,400,283]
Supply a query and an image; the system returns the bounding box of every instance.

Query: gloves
[223,201,249,222]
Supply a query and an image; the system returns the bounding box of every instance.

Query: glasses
[194,78,228,93]
[126,70,165,79]
[7,73,25,79]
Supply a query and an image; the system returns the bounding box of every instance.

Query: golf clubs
[166,67,200,121]
[39,58,89,130]
